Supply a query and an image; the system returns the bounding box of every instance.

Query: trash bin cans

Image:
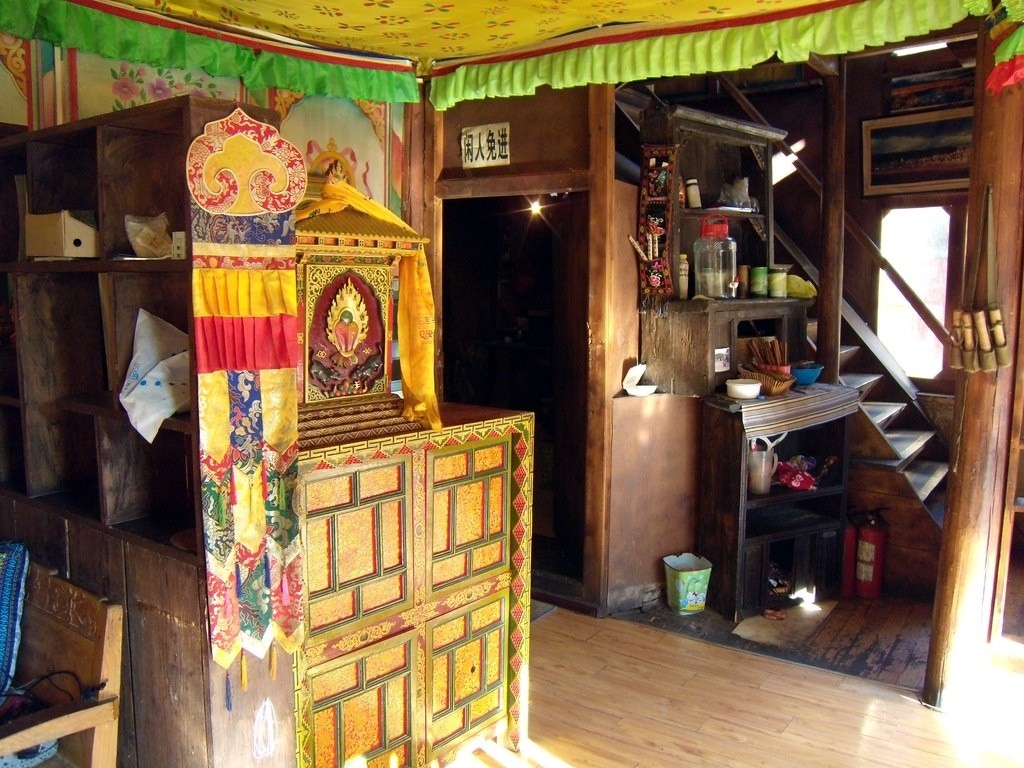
[662,552,712,615]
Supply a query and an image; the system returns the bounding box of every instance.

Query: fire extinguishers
[855,507,890,599]
[842,503,860,599]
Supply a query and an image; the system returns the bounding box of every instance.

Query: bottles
[679,254,689,300]
[686,178,702,209]
[693,214,737,298]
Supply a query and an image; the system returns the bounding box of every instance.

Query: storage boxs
[25,210,101,258]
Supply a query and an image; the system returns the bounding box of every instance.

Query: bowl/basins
[725,378,762,400]
[791,364,825,385]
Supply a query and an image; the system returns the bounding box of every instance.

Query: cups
[738,265,750,297]
[750,266,769,297]
[758,364,791,393]
[748,449,778,495]
[769,268,787,298]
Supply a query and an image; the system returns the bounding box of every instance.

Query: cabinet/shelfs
[695,382,859,623]
[639,105,815,394]
[0,94,294,768]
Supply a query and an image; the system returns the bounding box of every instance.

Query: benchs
[0,561,124,768]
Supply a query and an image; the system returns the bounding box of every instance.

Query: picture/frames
[858,101,974,201]
